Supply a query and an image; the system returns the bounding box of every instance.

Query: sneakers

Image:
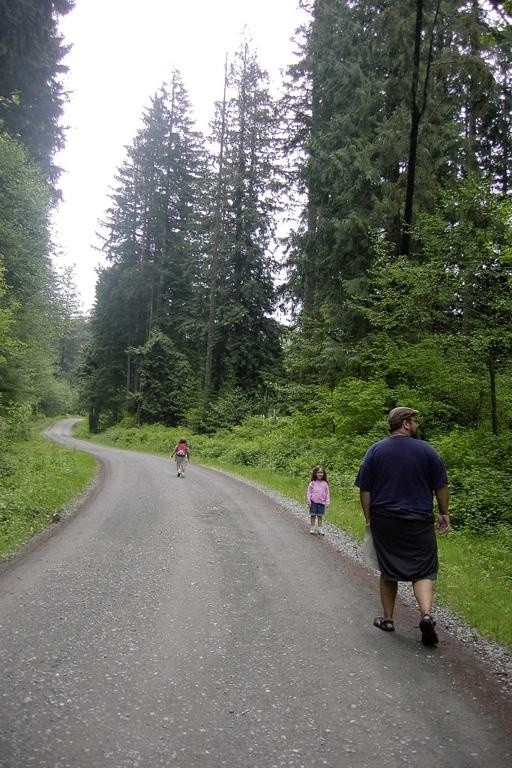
[181,473,184,478]
[318,526,325,535]
[177,471,181,477]
[310,526,317,534]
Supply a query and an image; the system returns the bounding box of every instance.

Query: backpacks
[175,443,187,456]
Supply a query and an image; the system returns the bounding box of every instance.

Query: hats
[388,407,419,427]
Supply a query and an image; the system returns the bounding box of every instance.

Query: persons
[170,438,191,478]
[354,407,450,645]
[305,466,331,536]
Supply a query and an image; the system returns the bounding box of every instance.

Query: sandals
[419,614,438,648]
[373,616,394,633]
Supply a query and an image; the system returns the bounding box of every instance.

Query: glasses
[407,420,418,424]
